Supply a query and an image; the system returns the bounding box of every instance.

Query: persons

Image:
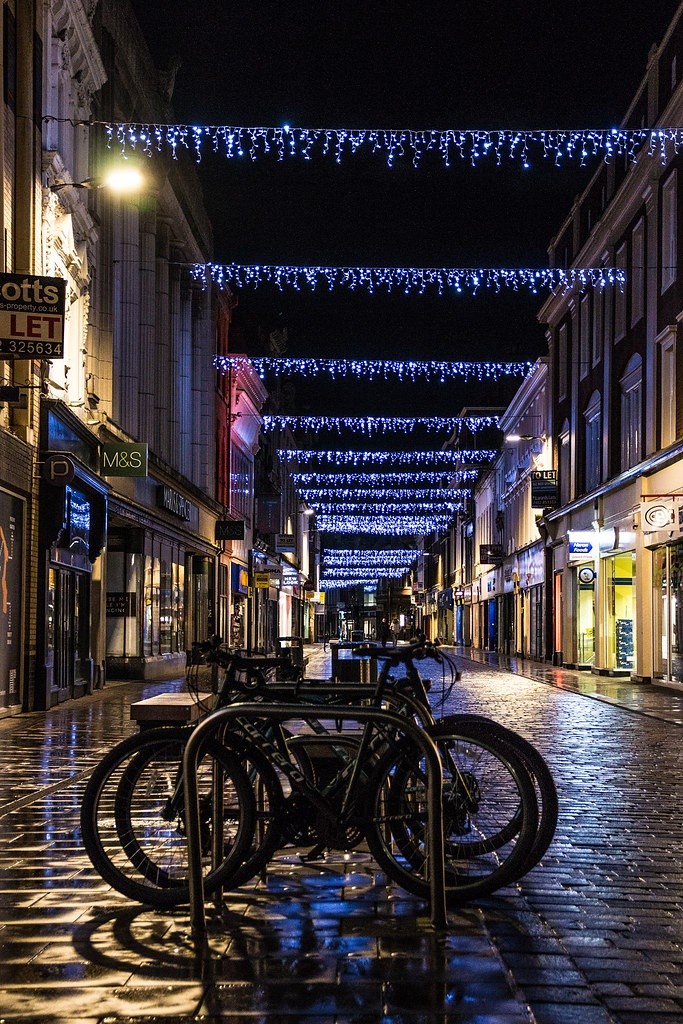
[338,626,342,641]
[389,617,401,648]
[378,618,388,648]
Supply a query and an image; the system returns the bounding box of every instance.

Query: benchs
[130,690,215,735]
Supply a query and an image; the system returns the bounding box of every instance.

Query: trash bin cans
[273,636,304,679]
[330,640,379,707]
[352,630,364,640]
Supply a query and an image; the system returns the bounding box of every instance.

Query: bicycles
[81,630,561,911]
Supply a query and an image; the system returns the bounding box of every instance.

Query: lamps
[50,175,108,193]
